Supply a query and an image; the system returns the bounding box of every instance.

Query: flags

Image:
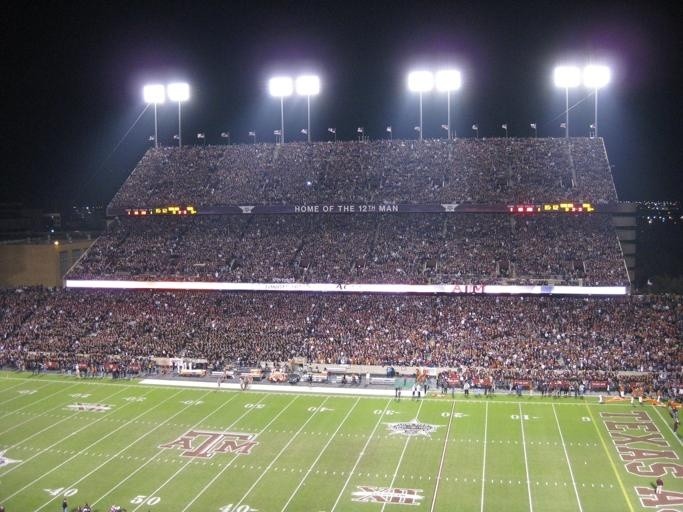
[247,131,256,137]
[440,124,450,130]
[559,122,567,128]
[298,128,310,135]
[327,128,336,134]
[271,129,281,137]
[413,126,422,132]
[470,123,479,130]
[530,123,536,129]
[501,124,507,129]
[385,127,392,132]
[589,120,599,129]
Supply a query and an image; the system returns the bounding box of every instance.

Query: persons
[0,133,682,406]
[672,406,680,423]
[655,475,664,494]
[0,504,4,512]
[56,497,126,512]
